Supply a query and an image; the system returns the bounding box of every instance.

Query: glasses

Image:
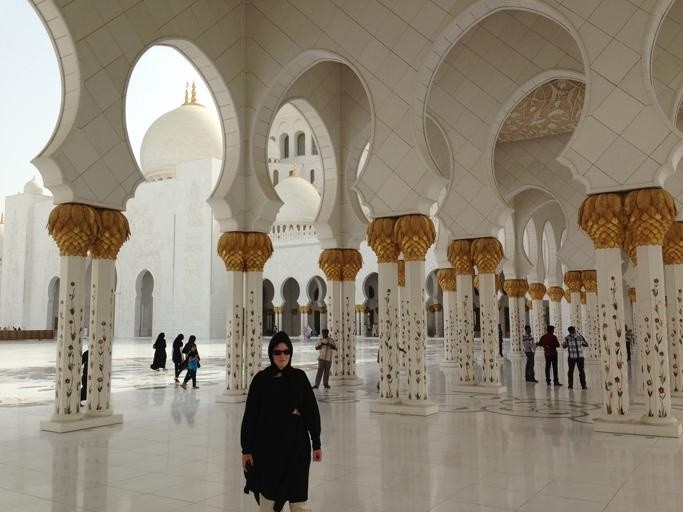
[274,349,291,356]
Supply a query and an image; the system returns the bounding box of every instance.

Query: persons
[561,325,588,391]
[238,329,323,511]
[623,323,635,362]
[520,324,539,382]
[148,331,168,371]
[170,333,184,383]
[310,328,337,390]
[179,335,200,390]
[497,323,504,357]
[537,324,563,387]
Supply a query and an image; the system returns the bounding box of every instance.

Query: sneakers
[324,385,330,388]
[312,385,318,389]
[582,386,587,390]
[547,382,551,385]
[174,378,199,390]
[568,385,573,389]
[526,377,539,383]
[554,382,563,386]
[156,368,168,371]
